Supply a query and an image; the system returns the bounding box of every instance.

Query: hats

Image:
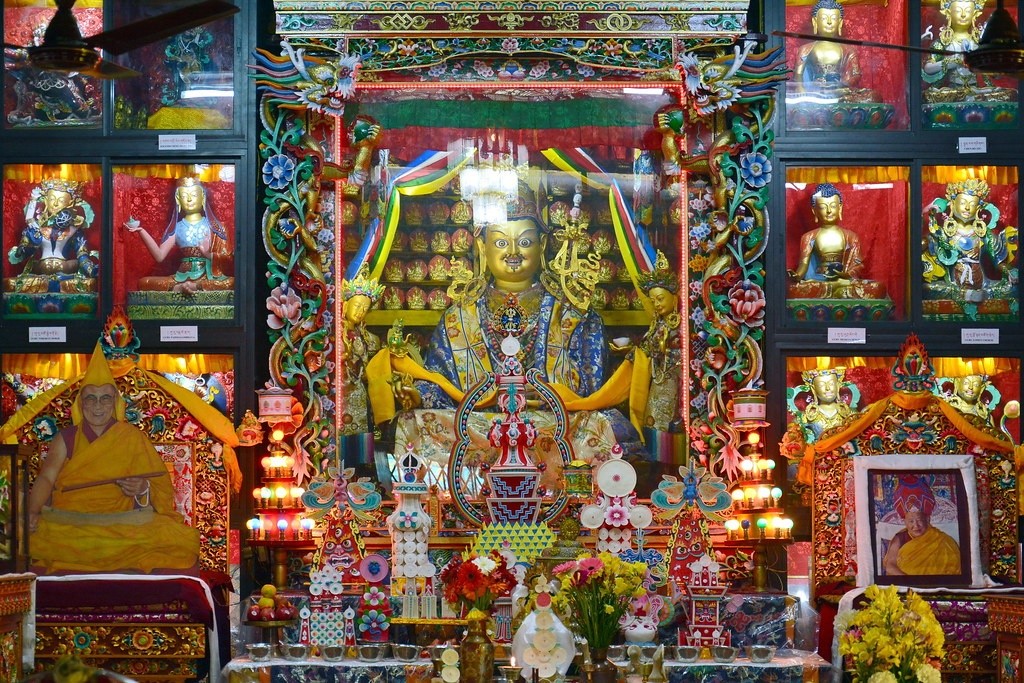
[70,341,126,426]
[894,474,935,519]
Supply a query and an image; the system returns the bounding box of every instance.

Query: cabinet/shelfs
[751,4,1022,508]
[1,1,268,472]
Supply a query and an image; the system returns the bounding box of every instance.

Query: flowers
[549,552,648,664]
[437,549,519,612]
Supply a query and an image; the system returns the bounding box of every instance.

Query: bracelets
[134,479,151,508]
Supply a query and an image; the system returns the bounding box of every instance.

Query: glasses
[82,396,113,407]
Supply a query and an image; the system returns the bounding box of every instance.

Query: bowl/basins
[245,643,423,662]
[606,645,776,663]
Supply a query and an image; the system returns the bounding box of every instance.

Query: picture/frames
[849,452,990,594]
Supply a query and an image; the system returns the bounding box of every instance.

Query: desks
[220,661,434,682]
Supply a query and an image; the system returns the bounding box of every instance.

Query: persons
[338,290,381,442]
[921,0,1020,108]
[789,0,883,110]
[154,19,222,111]
[937,370,1002,436]
[920,172,1020,307]
[387,211,655,488]
[10,18,94,122]
[121,178,233,297]
[791,363,852,447]
[607,285,683,427]
[26,379,203,575]
[13,179,99,282]
[785,182,889,304]
[884,508,961,576]
